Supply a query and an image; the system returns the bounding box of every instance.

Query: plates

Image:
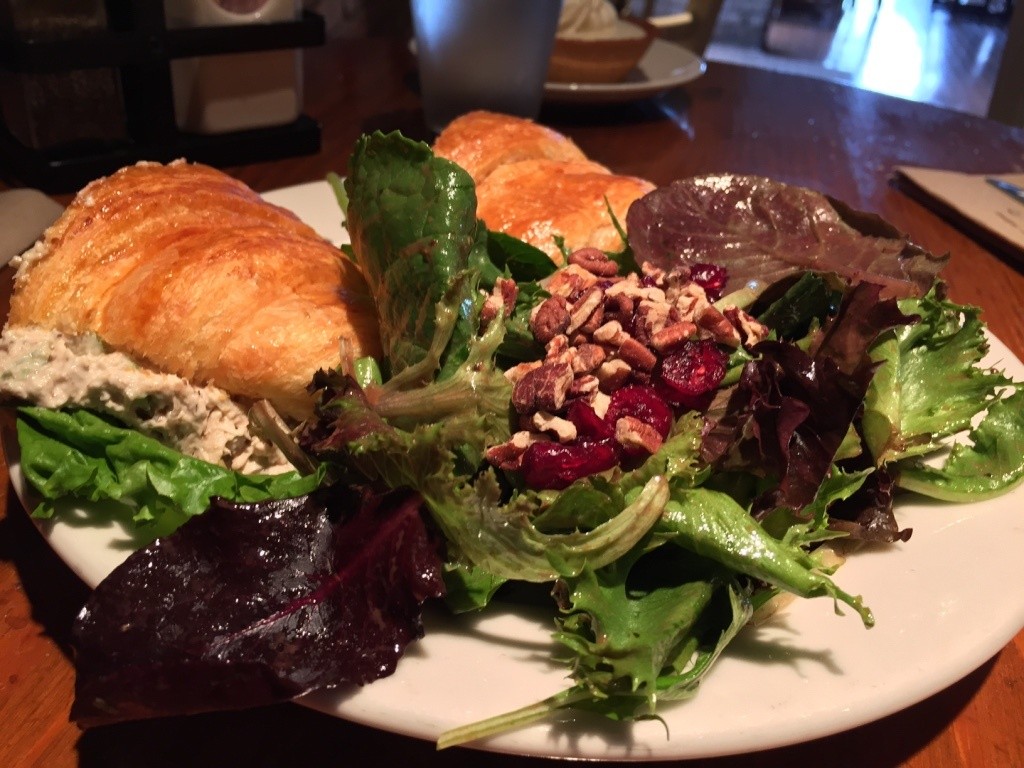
[7,175,1024,761]
[545,40,707,103]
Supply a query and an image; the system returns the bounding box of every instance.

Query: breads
[429,109,660,263]
[10,158,385,420]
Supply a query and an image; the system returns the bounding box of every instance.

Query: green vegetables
[15,124,1024,755]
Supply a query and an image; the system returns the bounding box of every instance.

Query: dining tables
[1,62,1024,766]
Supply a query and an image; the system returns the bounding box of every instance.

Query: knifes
[986,179,1024,203]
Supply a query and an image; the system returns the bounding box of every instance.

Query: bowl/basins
[547,14,658,84]
